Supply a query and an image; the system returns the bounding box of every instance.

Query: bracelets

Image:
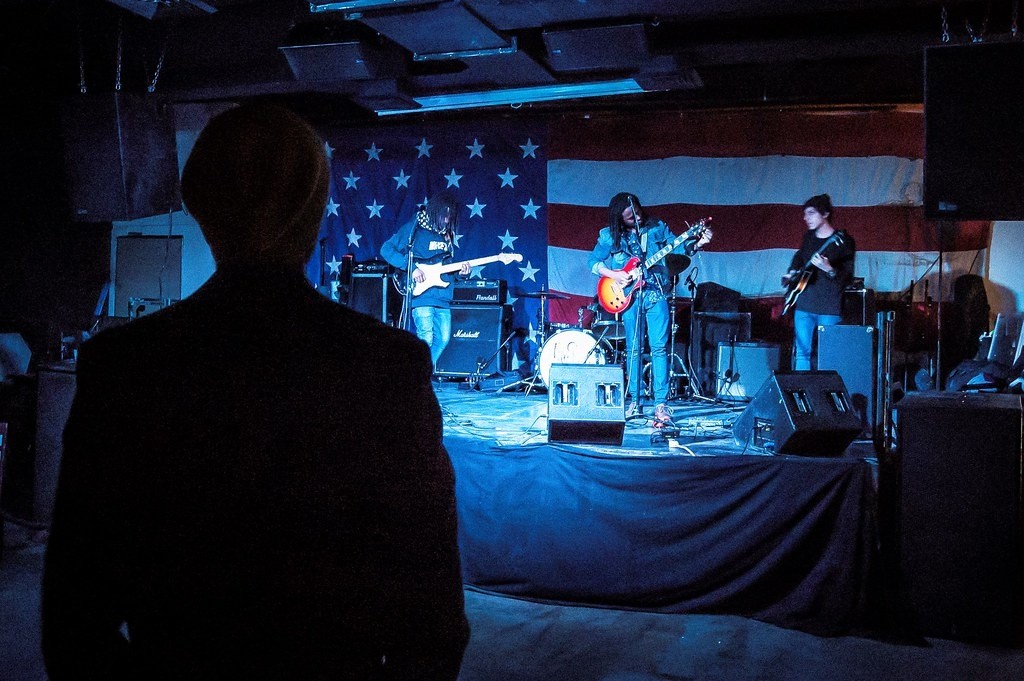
[696,243,702,249]
[827,268,836,279]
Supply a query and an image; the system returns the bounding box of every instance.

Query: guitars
[597,219,711,314]
[393,253,523,298]
[782,230,845,315]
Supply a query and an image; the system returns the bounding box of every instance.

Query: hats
[181,105,328,266]
[803,194,833,224]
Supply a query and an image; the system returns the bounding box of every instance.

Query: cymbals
[523,290,570,300]
[667,254,691,277]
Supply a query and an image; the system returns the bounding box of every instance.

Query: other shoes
[654,402,674,426]
[625,402,643,415]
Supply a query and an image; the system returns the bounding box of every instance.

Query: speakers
[545,363,626,446]
[817,324,876,438]
[716,341,785,401]
[732,369,864,457]
[923,39,1024,222]
[431,305,512,379]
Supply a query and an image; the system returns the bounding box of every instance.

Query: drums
[544,322,579,342]
[592,294,626,340]
[537,329,607,390]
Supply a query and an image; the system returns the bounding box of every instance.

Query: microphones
[719,368,732,393]
[476,356,482,374]
[627,195,634,204]
[684,268,695,285]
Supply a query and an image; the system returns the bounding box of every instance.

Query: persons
[43,106,471,681]
[781,193,856,370]
[588,192,713,421]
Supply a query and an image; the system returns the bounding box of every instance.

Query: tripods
[495,296,547,398]
[625,201,733,430]
